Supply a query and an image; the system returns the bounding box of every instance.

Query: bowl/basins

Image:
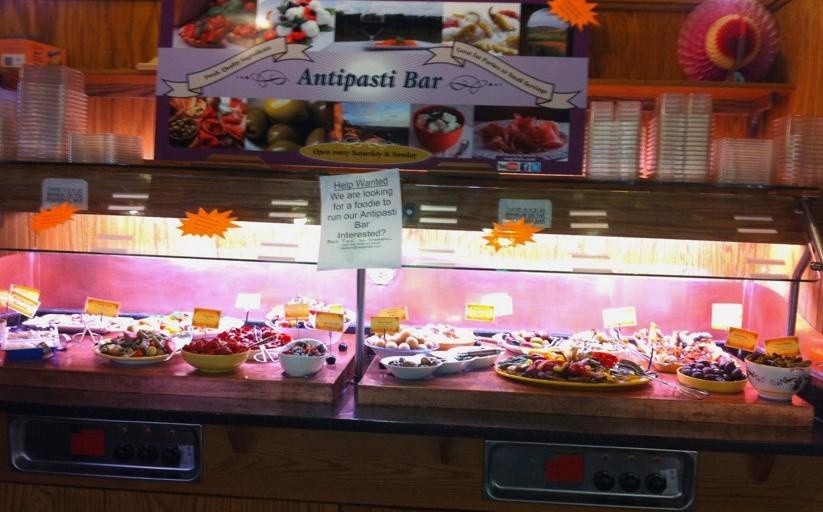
[378,356,440,380]
[412,105,465,152]
[168,119,198,145]
[744,348,811,403]
[180,346,251,372]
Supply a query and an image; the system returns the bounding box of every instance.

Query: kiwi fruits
[245,99,333,153]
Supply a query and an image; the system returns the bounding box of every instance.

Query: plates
[279,339,326,376]
[429,351,475,374]
[266,315,355,344]
[677,366,746,393]
[448,346,505,367]
[3,327,61,350]
[247,341,292,362]
[470,119,569,162]
[438,336,477,351]
[495,334,562,352]
[493,362,657,389]
[22,314,133,333]
[133,316,244,336]
[364,335,439,356]
[652,360,679,374]
[93,345,183,361]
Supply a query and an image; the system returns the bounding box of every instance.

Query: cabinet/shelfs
[0,70,823,510]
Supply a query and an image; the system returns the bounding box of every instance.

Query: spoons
[164,332,193,362]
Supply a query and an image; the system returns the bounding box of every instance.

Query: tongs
[72,313,102,345]
[620,359,707,397]
[254,326,279,363]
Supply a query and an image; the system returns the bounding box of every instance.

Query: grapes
[681,357,746,382]
